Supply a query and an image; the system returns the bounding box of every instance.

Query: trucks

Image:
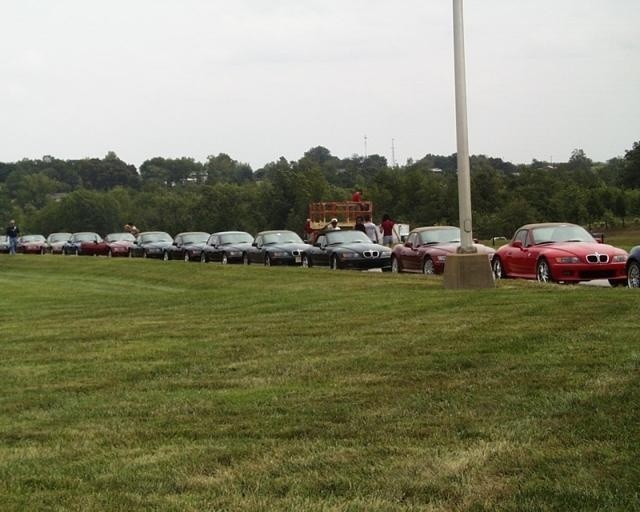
[305,201,384,250]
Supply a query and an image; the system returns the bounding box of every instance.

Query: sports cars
[390,224,497,277]
[298,228,392,271]
[488,222,626,288]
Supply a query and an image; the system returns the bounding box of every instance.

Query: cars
[491,236,507,240]
[624,245,639,288]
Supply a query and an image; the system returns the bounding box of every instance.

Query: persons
[124,223,130,233]
[352,190,368,209]
[6,219,19,255]
[129,223,141,236]
[303,214,400,250]
